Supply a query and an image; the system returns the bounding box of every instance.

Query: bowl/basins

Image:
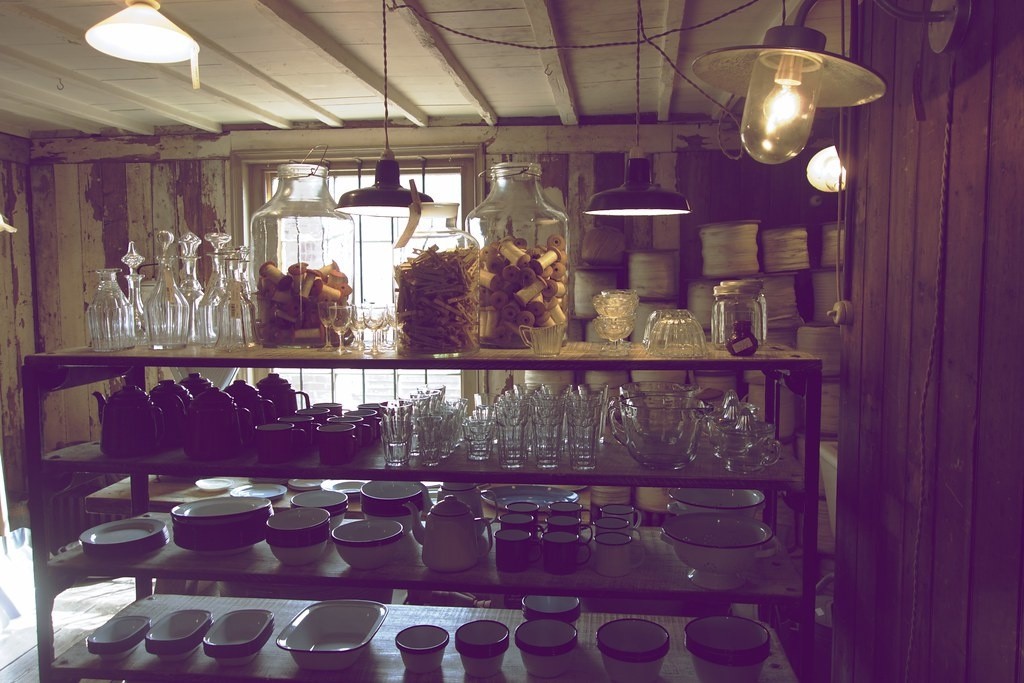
[85,598,772,683]
[657,488,777,592]
[642,308,709,359]
[264,478,424,573]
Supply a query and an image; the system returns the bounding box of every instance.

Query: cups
[519,322,566,357]
[251,382,782,476]
[592,288,640,357]
[491,501,643,580]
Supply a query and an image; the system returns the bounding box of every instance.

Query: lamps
[334,0,433,218]
[693,1,972,165]
[83,0,200,64]
[582,1,691,217]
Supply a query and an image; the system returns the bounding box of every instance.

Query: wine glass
[316,299,400,356]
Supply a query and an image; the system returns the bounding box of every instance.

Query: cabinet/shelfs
[22,342,822,683]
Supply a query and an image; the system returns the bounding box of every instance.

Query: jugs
[399,481,499,574]
[92,371,311,462]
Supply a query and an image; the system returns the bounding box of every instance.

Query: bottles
[86,164,362,354]
[710,278,768,356]
[393,201,483,357]
[463,161,574,349]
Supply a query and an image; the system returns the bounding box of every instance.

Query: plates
[481,485,581,513]
[79,476,374,572]
